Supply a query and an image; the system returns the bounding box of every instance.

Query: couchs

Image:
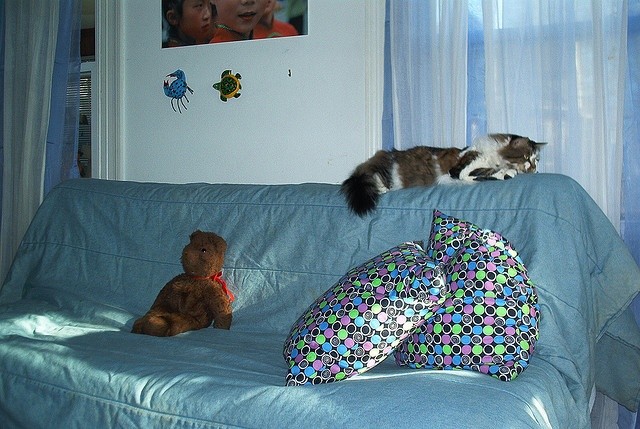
[1,174,638,429]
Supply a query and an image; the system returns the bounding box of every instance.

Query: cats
[337,134,548,218]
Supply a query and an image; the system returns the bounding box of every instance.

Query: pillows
[388,205,540,383]
[283,237,449,388]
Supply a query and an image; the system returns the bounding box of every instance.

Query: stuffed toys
[132,229,232,337]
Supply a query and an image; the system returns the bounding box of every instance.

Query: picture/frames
[158,1,310,51]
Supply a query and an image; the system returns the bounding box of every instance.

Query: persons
[258,0,298,37]
[209,0,284,45]
[162,0,213,47]
